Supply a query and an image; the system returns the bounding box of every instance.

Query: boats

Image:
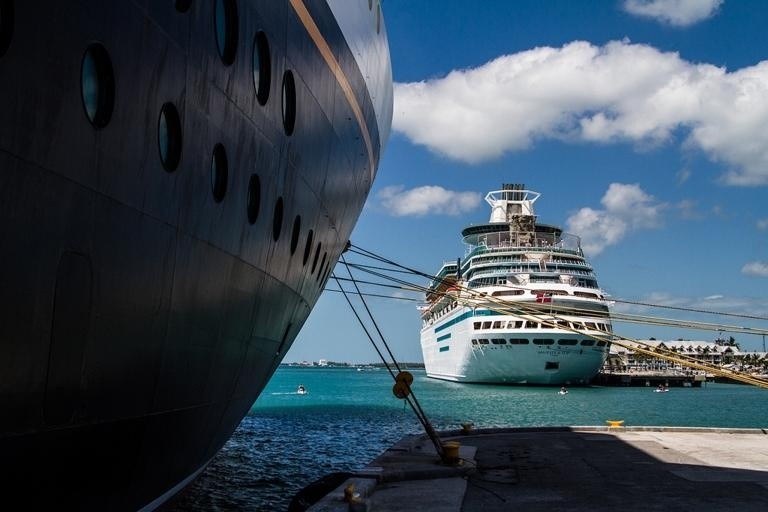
[298,384,306,394]
[559,387,568,395]
[653,386,670,393]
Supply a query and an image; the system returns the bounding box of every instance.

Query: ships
[417,184,614,386]
[0,1,396,512]
